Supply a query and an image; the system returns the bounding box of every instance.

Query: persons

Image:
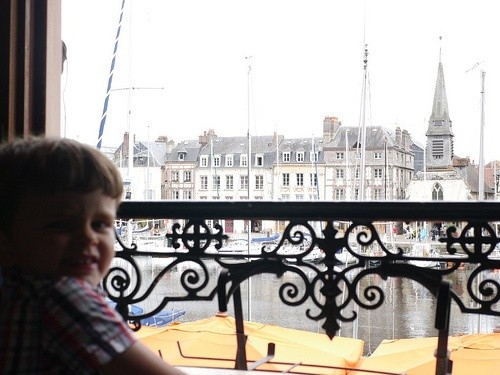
[386,223,450,240]
[0,138,188,375]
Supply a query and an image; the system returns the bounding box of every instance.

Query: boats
[114,230,442,269]
